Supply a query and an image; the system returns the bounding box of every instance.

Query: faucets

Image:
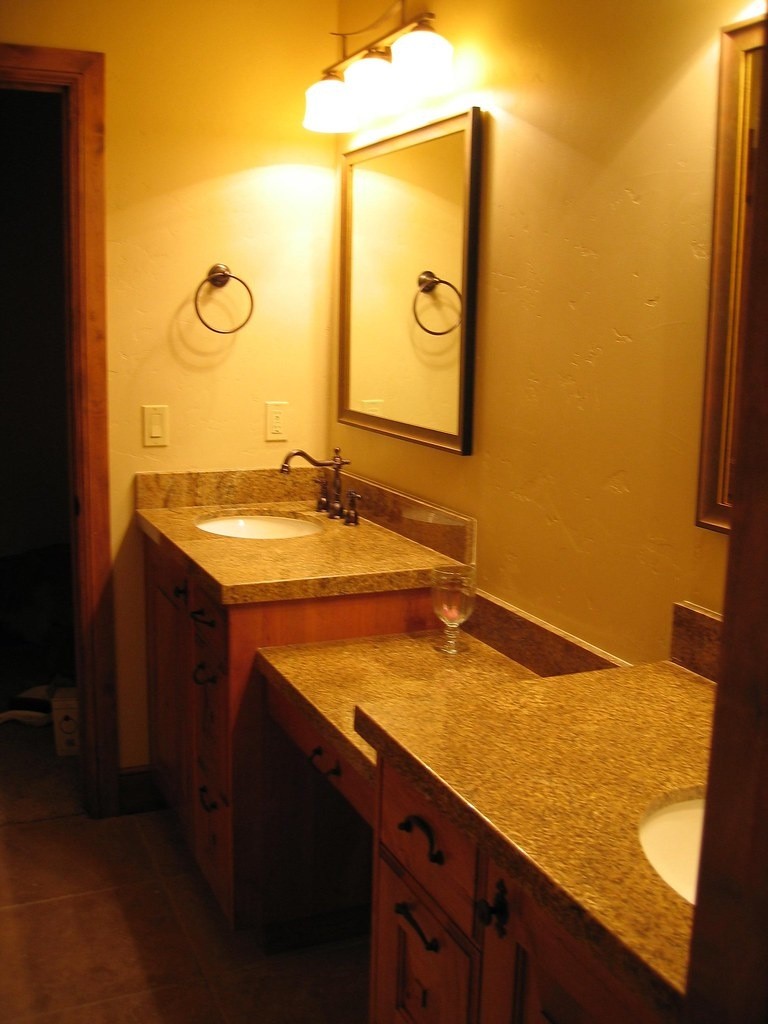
[280,447,350,519]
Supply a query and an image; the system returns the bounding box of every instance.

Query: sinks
[639,784,706,905]
[194,508,325,539]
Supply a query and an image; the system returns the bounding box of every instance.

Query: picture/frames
[695,13,768,539]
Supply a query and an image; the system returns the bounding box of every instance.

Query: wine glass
[432,564,478,655]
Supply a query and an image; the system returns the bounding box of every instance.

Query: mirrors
[337,106,483,457]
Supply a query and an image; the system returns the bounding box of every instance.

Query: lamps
[265,400,289,442]
[302,12,457,134]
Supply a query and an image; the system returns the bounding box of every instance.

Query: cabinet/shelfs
[139,530,472,936]
[368,749,671,1024]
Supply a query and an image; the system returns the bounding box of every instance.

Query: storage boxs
[50,688,86,757]
